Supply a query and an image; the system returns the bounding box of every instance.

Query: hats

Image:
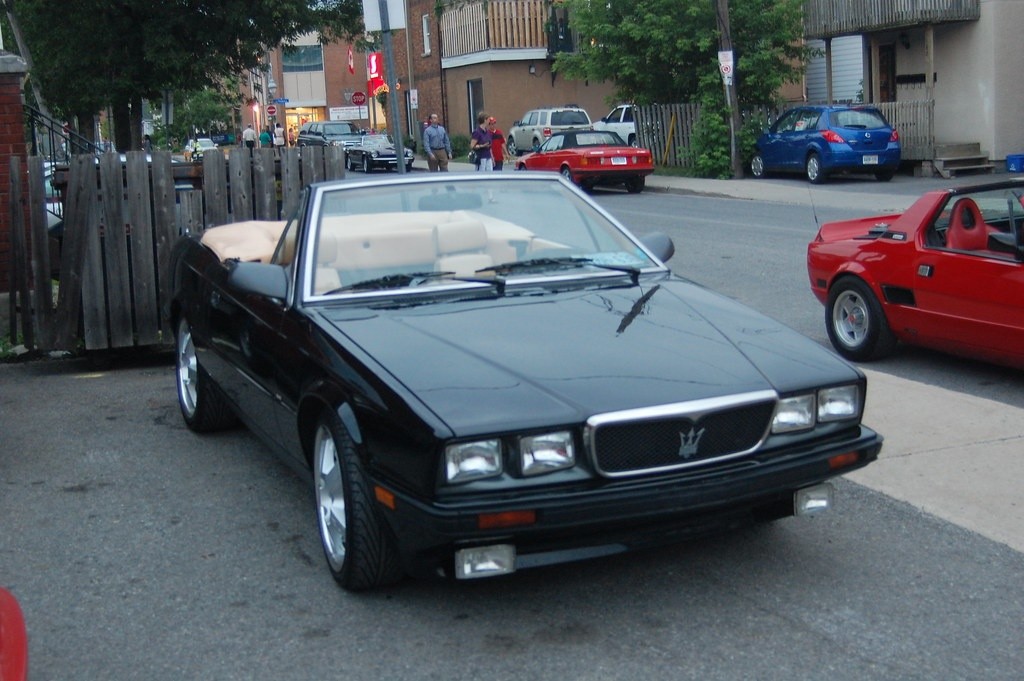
[488,118,496,123]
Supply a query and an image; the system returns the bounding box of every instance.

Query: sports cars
[348,134,416,173]
[165,168,885,595]
[806,176,1024,369]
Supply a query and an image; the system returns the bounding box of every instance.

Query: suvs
[295,121,362,158]
[505,105,593,157]
[593,103,661,148]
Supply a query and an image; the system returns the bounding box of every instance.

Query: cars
[185,138,219,162]
[514,129,654,193]
[747,104,900,182]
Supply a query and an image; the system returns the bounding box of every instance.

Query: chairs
[945,197,987,251]
[285,231,341,293]
[432,220,493,278]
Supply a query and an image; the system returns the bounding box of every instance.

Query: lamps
[529,63,535,74]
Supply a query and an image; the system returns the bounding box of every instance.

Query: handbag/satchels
[469,152,480,164]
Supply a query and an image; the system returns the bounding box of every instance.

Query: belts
[430,147,446,150]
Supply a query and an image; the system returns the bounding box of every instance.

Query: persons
[191,137,201,160]
[289,128,296,141]
[487,116,511,171]
[259,128,271,148]
[469,111,496,202]
[242,123,257,157]
[103,136,110,153]
[370,128,377,135]
[142,134,152,151]
[264,125,273,147]
[274,122,286,147]
[423,114,452,171]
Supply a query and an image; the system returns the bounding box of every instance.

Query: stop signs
[268,106,276,115]
[351,90,366,105]
